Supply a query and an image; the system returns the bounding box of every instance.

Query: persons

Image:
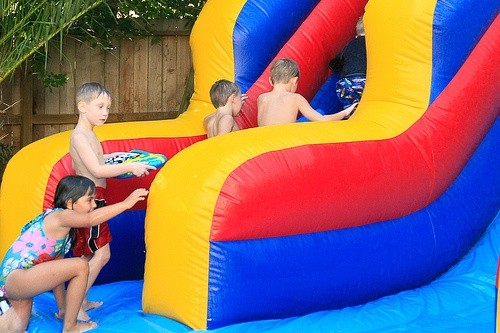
[69,82,157,321]
[327,12,366,115]
[257,57,360,128]
[203,79,248,139]
[0,175,150,333]
